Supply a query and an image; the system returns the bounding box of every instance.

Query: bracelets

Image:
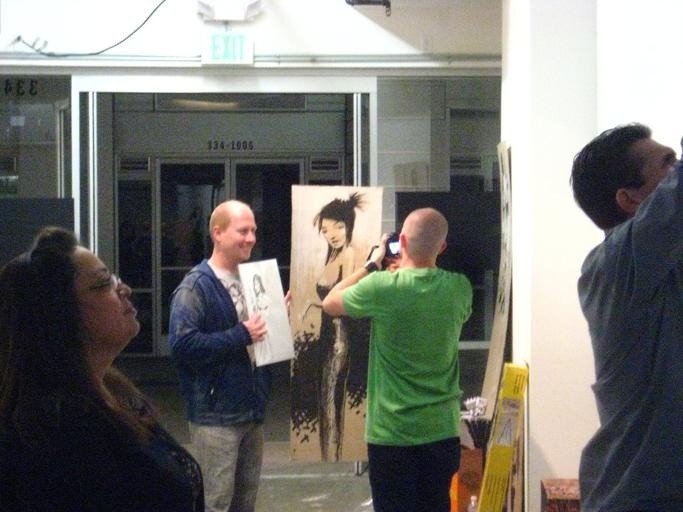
[366,260,378,273]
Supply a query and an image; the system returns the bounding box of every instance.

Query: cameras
[385,231,400,259]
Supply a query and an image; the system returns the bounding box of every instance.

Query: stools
[541,477,582,511]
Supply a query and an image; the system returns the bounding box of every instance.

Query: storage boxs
[454,446,484,511]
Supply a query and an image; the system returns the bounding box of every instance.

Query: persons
[312,191,366,465]
[167,200,291,512]
[570,124,683,512]
[0,224,206,512]
[322,206,474,512]
[252,270,278,362]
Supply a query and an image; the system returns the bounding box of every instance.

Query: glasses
[75,273,121,293]
